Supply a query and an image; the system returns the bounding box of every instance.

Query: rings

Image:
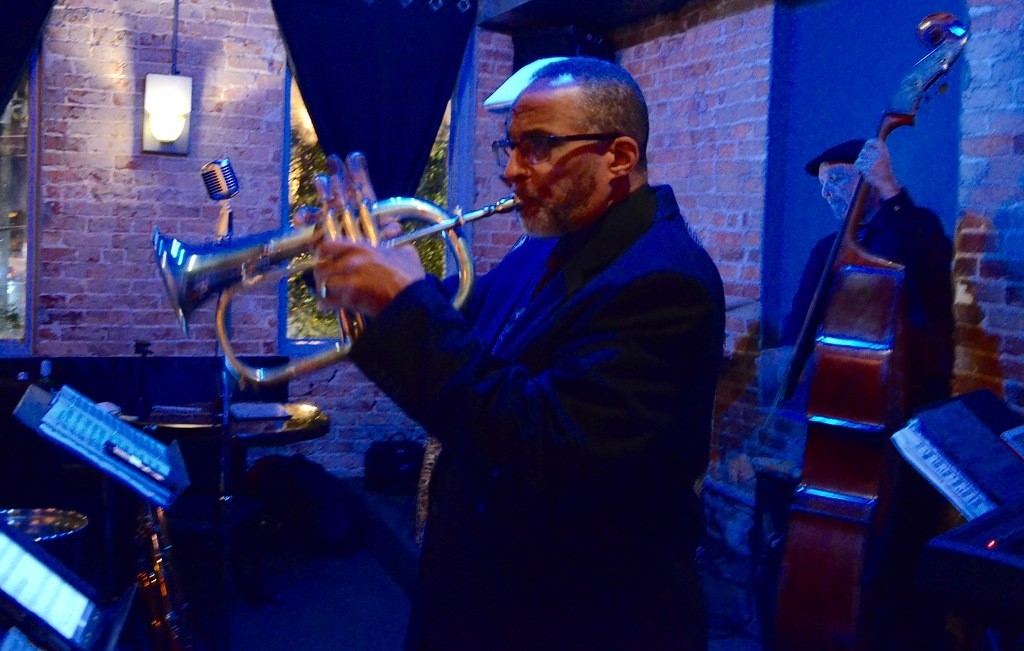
[318,278,329,300]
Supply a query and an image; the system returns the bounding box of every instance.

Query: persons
[315,58,726,651]
[784,138,969,651]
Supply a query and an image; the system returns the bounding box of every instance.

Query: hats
[804,138,867,177]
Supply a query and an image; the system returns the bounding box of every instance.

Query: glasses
[490,131,618,167]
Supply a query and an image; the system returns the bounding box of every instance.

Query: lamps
[143,74,192,155]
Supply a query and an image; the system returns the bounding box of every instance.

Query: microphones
[201,158,239,202]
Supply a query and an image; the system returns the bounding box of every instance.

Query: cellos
[767,10,970,651]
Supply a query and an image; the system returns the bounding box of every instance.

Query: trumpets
[148,194,524,385]
